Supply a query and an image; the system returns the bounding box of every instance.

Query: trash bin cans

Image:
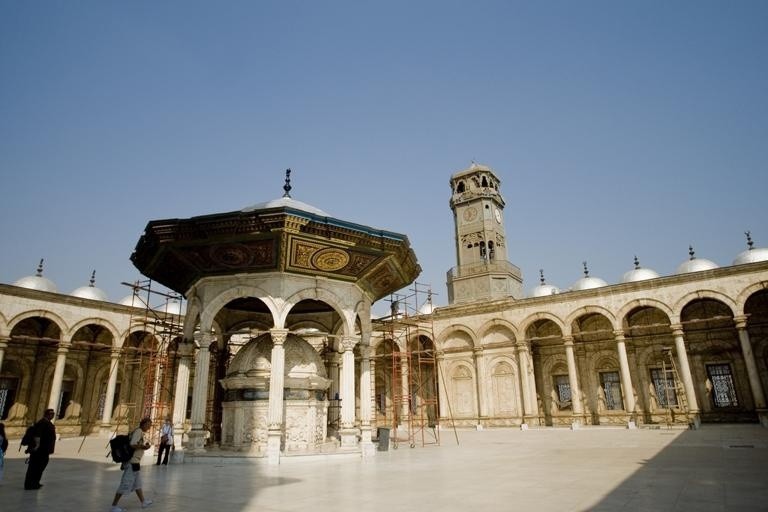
[377,427,390,451]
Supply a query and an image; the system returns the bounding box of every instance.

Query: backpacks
[109,428,141,463]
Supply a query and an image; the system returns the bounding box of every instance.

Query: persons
[155,418,173,465]
[21,409,56,490]
[0,423,9,486]
[107,417,152,512]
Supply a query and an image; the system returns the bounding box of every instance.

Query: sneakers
[110,506,125,512]
[142,500,152,508]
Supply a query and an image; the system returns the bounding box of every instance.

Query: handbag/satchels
[161,434,169,445]
[0,434,8,451]
[22,425,35,445]
